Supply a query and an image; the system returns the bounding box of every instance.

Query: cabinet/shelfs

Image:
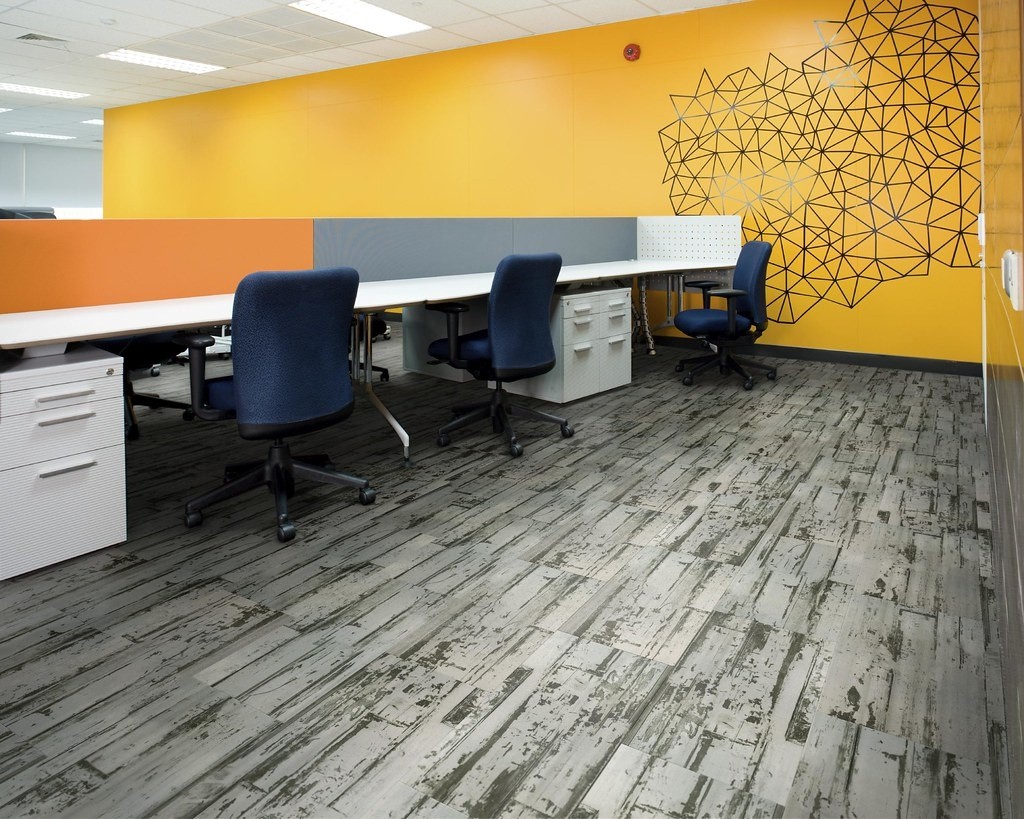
[489,286,631,408]
[402,301,488,384]
[0,345,127,589]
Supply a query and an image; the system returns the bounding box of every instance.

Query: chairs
[674,240,778,390]
[178,265,375,541]
[63,331,193,439]
[347,310,390,383]
[424,251,575,455]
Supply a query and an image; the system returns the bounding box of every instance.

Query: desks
[0,259,739,466]
